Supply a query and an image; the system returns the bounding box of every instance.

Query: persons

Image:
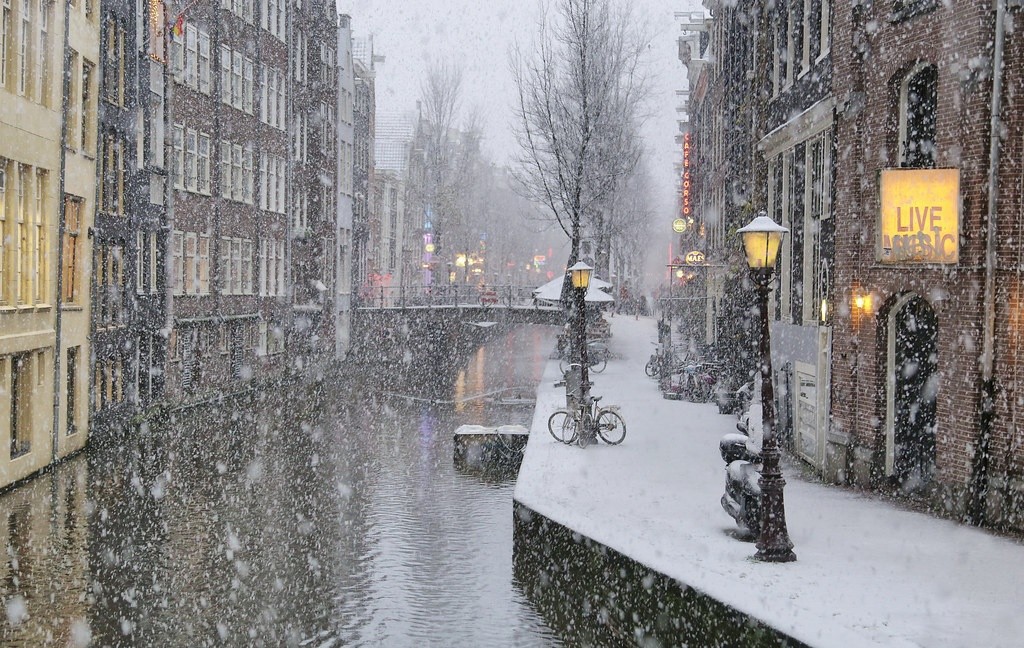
[615,281,630,316]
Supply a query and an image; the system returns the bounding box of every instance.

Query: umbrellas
[535,282,614,302]
[533,274,612,294]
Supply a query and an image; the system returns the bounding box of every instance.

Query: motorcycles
[719,431,762,544]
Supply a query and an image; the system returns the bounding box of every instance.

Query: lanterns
[685,252,706,266]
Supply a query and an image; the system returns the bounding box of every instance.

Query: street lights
[567,261,595,451]
[457,253,467,309]
[424,243,435,304]
[525,263,532,310]
[677,263,684,355]
[738,213,790,564]
[445,259,453,302]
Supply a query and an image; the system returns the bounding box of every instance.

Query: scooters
[676,364,713,404]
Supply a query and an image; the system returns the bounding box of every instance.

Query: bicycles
[560,334,606,373]
[644,339,720,378]
[547,391,626,445]
[477,284,530,308]
